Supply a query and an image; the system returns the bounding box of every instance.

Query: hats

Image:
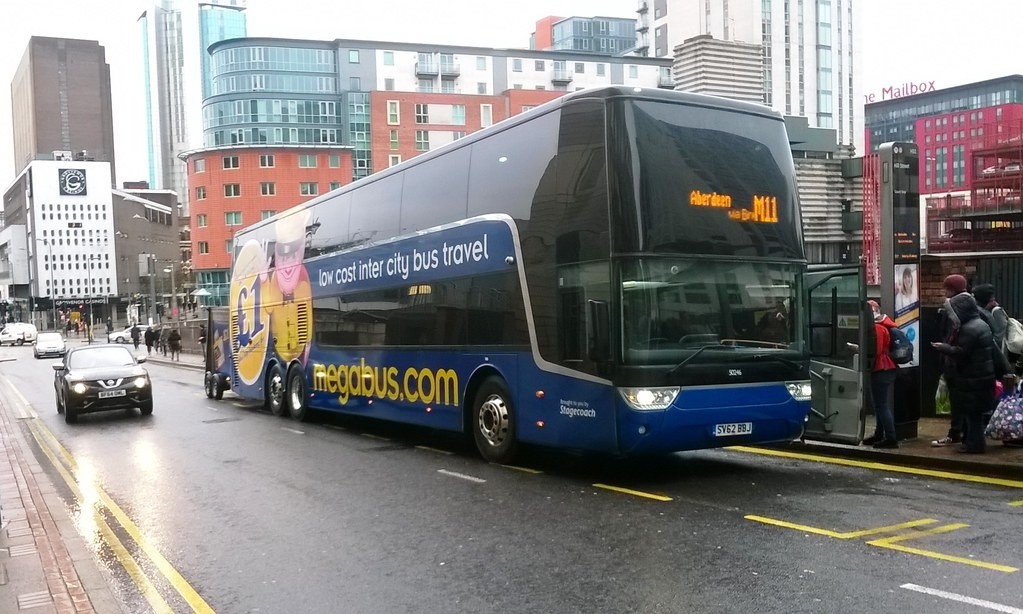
[943,274,967,292]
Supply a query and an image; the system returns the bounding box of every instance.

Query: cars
[32,332,66,359]
[52,344,153,423]
[0,323,38,346]
[109,325,150,344]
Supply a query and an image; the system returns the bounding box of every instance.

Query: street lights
[35,238,56,331]
[123,198,158,324]
[1,257,16,322]
[88,257,101,342]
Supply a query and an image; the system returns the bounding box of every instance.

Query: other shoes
[863,431,884,445]
[872,439,899,449]
[931,437,962,447]
[958,447,984,454]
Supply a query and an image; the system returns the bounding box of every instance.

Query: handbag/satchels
[993,340,1012,378]
[984,396,1023,442]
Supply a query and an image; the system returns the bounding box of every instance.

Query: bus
[227,84,869,457]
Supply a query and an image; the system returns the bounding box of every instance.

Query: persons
[639,310,718,342]
[62,300,206,362]
[895,268,918,310]
[776,297,808,346]
[867,275,1017,453]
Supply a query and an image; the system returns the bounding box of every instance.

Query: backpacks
[888,328,914,364]
[992,306,1023,354]
